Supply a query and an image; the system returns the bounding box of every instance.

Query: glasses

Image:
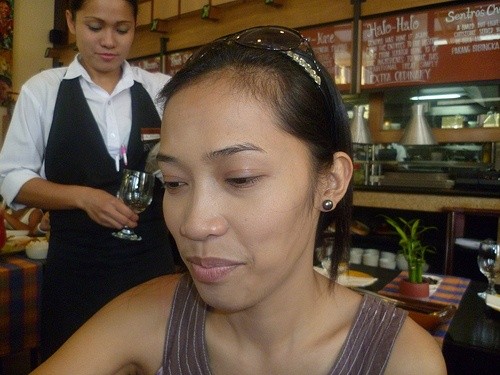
[180,25,336,121]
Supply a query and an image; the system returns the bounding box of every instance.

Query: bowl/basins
[349,247,407,270]
[24,242,49,260]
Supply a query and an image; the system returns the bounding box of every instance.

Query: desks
[442,207,500,275]
[316,261,500,357]
[0,234,42,374]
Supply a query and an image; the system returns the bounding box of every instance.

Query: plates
[382,293,457,330]
[484,294,500,310]
[5,230,29,240]
[314,266,378,287]
[0,235,46,255]
[401,160,457,167]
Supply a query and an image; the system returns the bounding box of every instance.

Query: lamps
[399,87,437,145]
[348,104,371,144]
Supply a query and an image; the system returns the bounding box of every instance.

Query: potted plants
[379,213,436,299]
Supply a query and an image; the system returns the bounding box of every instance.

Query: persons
[0,0,182,375]
[28,26,448,375]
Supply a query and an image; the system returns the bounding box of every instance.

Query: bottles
[0,194,7,249]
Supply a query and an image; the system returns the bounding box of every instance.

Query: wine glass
[112,168,155,241]
[477,240,500,301]
